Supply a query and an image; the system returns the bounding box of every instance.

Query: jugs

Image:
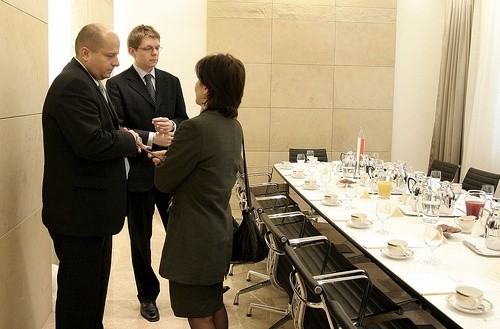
[369,173,393,200]
[479,206,500,251]
[455,216,476,234]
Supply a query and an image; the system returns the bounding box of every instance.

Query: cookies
[436,224,462,233]
[338,179,357,184]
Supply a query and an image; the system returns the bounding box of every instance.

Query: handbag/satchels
[232,208,269,264]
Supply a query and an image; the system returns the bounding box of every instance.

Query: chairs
[288,148,329,162]
[227,155,436,329]
[427,159,457,184]
[461,167,500,197]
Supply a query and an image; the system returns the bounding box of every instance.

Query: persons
[148,53,246,329]
[106,25,230,323]
[42,23,143,329]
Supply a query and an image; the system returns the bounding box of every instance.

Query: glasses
[137,45,162,52]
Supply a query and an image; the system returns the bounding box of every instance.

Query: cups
[282,161,290,168]
[387,239,408,256]
[456,285,483,310]
[324,194,338,204]
[340,151,494,225]
[293,170,303,177]
[305,179,317,188]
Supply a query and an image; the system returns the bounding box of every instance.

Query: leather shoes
[141,301,159,321]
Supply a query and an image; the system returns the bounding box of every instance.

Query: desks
[273,160,500,329]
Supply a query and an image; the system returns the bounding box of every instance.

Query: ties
[98,81,108,102]
[144,74,156,102]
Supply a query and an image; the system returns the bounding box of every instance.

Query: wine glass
[375,201,392,234]
[297,149,332,192]
[344,183,358,211]
[424,226,443,265]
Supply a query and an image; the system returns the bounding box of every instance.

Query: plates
[301,184,320,190]
[380,247,414,260]
[321,199,342,206]
[291,175,307,179]
[346,220,373,230]
[280,165,293,170]
[446,294,494,314]
